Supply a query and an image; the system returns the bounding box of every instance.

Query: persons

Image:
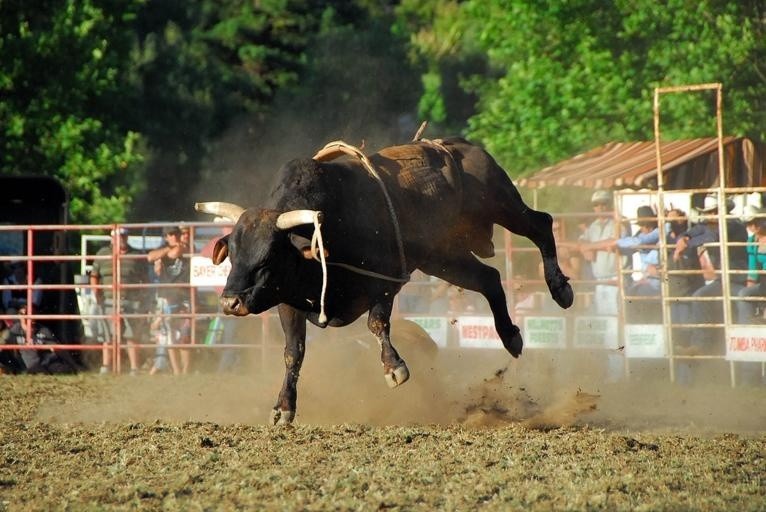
[1,183,766,390]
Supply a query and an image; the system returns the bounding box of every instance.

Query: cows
[191,134,574,426]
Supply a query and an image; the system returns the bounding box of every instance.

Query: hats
[591,191,610,203]
[696,196,735,213]
[111,228,128,236]
[632,206,657,225]
[164,227,181,234]
[738,205,766,224]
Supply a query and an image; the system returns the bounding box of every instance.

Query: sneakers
[677,346,703,355]
